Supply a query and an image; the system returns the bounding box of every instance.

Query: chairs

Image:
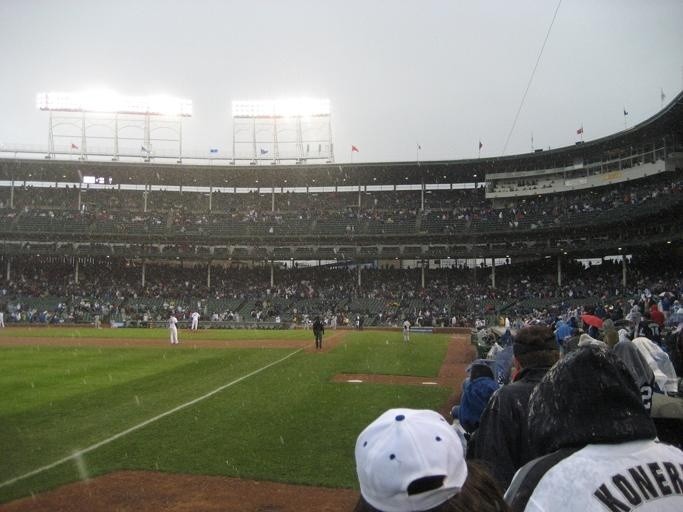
[0,134,683,330]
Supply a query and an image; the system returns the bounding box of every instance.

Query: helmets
[466,359,509,388]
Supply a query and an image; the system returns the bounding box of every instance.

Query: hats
[354,407,468,512]
[460,378,500,434]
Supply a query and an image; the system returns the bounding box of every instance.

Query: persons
[313,318,325,351]
[0,128,683,320]
[504,343,683,511]
[191,310,201,331]
[404,321,411,341]
[169,315,179,345]
[94,314,101,329]
[329,313,339,329]
[0,312,6,329]
[354,407,510,511]
[454,321,682,489]
